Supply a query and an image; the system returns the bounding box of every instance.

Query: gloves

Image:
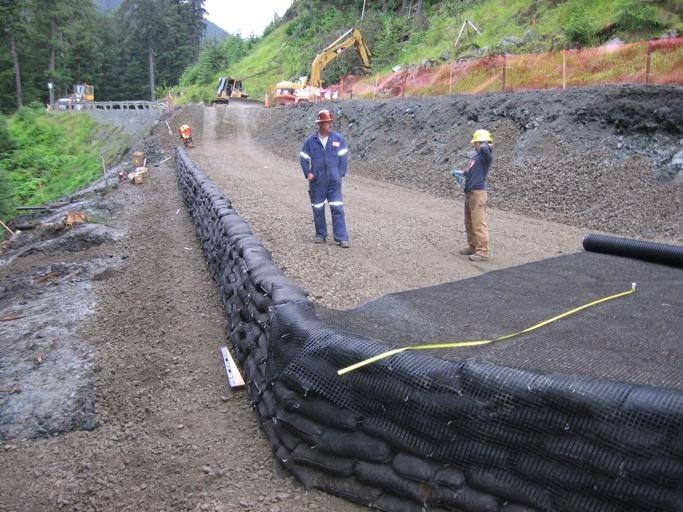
[451,171,462,183]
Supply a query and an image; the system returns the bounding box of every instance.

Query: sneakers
[468,253,489,261]
[338,240,350,248]
[460,247,472,255]
[313,235,327,243]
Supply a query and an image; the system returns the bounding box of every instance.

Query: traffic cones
[263,90,270,107]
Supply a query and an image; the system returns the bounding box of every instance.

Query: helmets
[314,109,336,122]
[470,129,494,143]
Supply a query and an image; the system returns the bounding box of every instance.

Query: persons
[178,123,194,148]
[298,110,351,248]
[450,130,492,262]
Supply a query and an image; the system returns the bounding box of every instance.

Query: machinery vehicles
[53,82,98,111]
[202,74,250,108]
[271,27,377,106]
